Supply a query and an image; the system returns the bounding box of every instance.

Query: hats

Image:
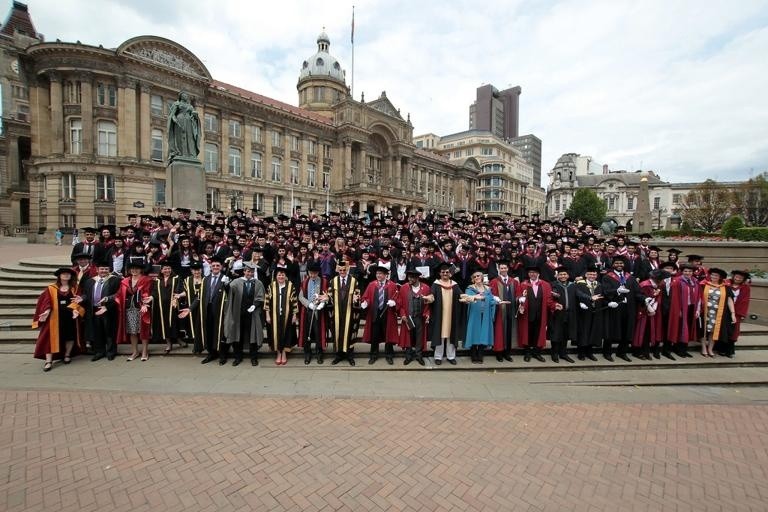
[75,228,125,269]
[125,207,606,278]
[606,224,752,280]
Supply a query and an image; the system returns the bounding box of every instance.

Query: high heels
[178,339,190,348]
[142,350,148,361]
[163,343,173,354]
[126,351,140,362]
[42,362,53,372]
[62,353,72,364]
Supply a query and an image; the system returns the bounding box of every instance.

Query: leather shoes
[304,356,458,365]
[201,350,218,365]
[250,357,258,367]
[274,352,287,365]
[91,353,105,361]
[218,354,228,365]
[106,352,117,361]
[470,352,598,364]
[231,358,243,366]
[604,349,693,363]
[701,350,715,357]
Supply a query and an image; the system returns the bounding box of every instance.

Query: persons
[166,91,202,159]
[30,205,751,369]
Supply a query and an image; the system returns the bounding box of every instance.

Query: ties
[311,280,318,303]
[246,280,250,295]
[689,282,696,305]
[378,283,384,310]
[93,280,105,305]
[590,283,596,308]
[342,280,346,295]
[532,282,538,299]
